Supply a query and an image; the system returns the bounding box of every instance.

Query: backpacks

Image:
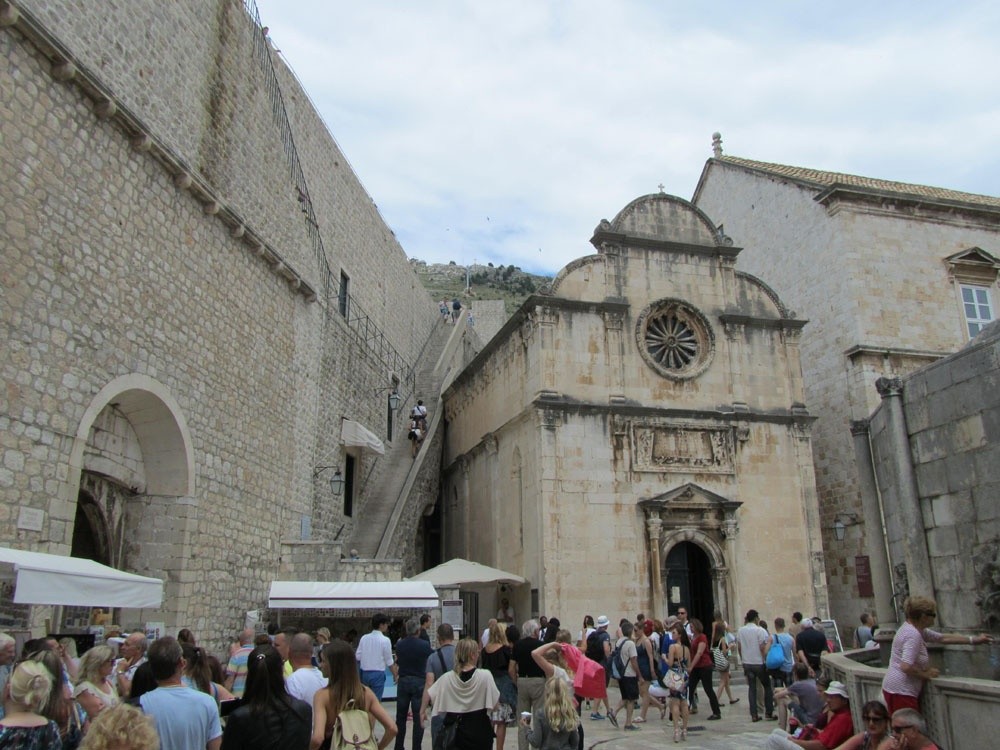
[609,639,631,679]
[326,684,379,750]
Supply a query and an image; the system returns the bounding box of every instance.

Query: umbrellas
[403,559,531,636]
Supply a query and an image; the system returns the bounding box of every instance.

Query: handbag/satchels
[663,670,685,692]
[711,639,729,671]
[765,634,784,669]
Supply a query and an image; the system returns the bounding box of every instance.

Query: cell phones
[219,698,243,718]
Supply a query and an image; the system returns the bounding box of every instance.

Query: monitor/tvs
[47,633,96,658]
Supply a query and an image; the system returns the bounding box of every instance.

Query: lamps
[376,381,401,410]
[830,513,857,541]
[313,465,346,495]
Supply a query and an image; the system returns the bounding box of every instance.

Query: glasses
[815,679,826,689]
[102,659,115,666]
[11,659,29,675]
[677,612,687,615]
[921,611,936,618]
[891,725,914,734]
[862,716,884,724]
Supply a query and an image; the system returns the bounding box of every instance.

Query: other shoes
[407,712,413,719]
[586,698,778,743]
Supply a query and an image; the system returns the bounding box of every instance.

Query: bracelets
[969,636,972,645]
[118,671,124,674]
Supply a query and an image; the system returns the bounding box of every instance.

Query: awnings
[341,419,385,455]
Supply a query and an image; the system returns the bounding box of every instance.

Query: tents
[269,582,438,632]
[0,548,164,634]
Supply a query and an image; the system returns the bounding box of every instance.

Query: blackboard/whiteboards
[821,620,843,653]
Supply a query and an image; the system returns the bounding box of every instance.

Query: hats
[664,616,681,630]
[598,616,609,628]
[108,633,130,643]
[823,681,850,699]
[22,639,53,661]
[642,620,653,636]
[316,627,330,642]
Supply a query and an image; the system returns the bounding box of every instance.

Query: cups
[521,710,532,725]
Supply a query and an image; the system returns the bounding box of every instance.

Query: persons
[882,595,995,732]
[853,613,879,648]
[408,399,427,458]
[496,598,514,622]
[438,297,461,323]
[0,607,854,750]
[832,701,940,750]
[341,549,360,559]
